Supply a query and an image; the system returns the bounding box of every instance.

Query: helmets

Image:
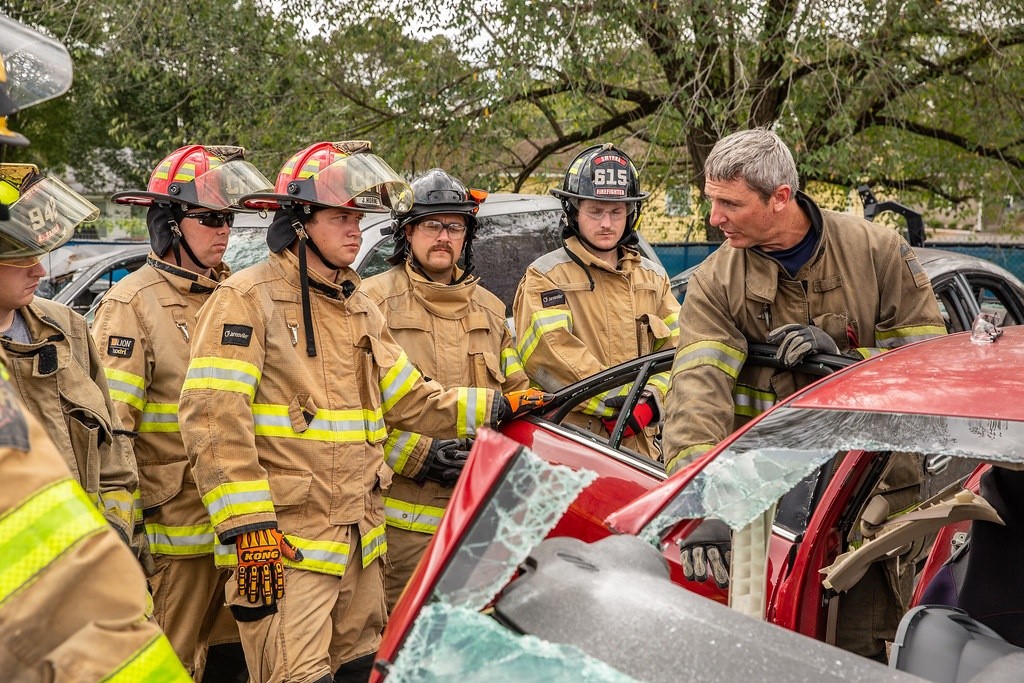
[548,143,651,202]
[238,140,391,213]
[392,167,478,230]
[1,173,74,259]
[111,145,242,211]
[0,52,31,147]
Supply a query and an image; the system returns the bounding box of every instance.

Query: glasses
[183,211,234,228]
[414,220,469,240]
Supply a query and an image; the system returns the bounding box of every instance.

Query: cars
[27,193,1024,683]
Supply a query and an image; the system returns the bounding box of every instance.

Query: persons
[662,128,949,667]
[513,141,681,465]
[0,6,557,683]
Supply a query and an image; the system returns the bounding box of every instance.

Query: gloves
[679,520,732,589]
[495,385,557,426]
[599,394,659,440]
[235,524,303,603]
[125,523,157,577]
[766,322,841,367]
[424,438,476,489]
